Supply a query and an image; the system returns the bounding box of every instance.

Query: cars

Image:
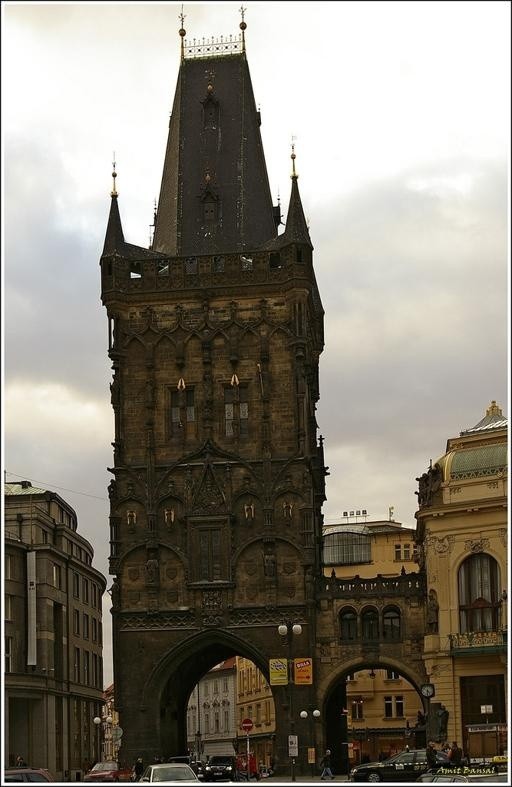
[137,756,240,782]
[84,761,135,782]
[418,772,508,783]
[349,749,466,782]
[4,768,53,783]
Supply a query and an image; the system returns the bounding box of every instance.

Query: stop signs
[242,719,253,731]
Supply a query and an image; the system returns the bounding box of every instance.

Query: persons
[447,741,462,768]
[426,740,437,768]
[135,757,143,776]
[16,756,26,767]
[319,749,335,780]
[83,758,93,773]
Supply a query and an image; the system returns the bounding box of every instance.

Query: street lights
[300,708,321,778]
[93,716,113,760]
[278,617,303,782]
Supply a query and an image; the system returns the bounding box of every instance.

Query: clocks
[420,683,435,697]
